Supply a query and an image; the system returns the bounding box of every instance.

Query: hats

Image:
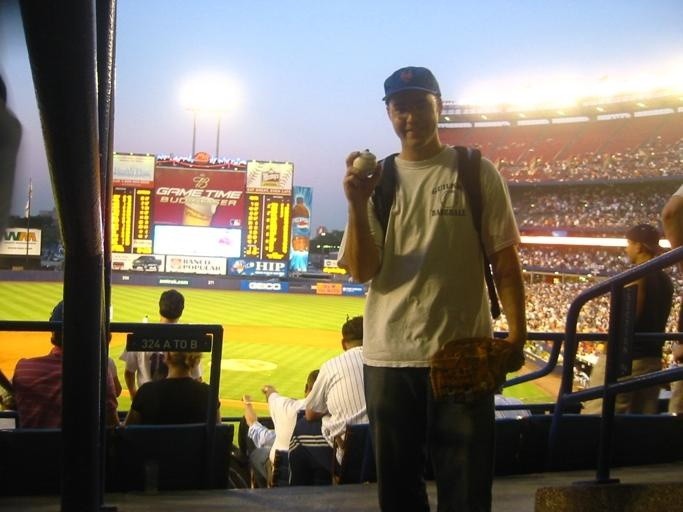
[382,66,441,101]
[626,224,664,253]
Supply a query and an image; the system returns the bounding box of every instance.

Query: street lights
[177,72,240,157]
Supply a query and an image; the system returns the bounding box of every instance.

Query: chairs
[1,413,682,490]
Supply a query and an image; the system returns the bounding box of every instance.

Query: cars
[132,256,161,272]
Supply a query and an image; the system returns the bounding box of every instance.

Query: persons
[489,245,683,376]
[493,135,683,181]
[602,183,682,414]
[509,182,680,229]
[336,67,527,512]
[11,300,122,428]
[346,313,349,322]
[118,290,222,426]
[244,315,371,485]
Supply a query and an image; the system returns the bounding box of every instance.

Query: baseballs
[353,152,377,178]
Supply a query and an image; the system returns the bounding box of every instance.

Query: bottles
[352,149,376,176]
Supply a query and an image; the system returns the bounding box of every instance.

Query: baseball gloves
[430,338,524,402]
